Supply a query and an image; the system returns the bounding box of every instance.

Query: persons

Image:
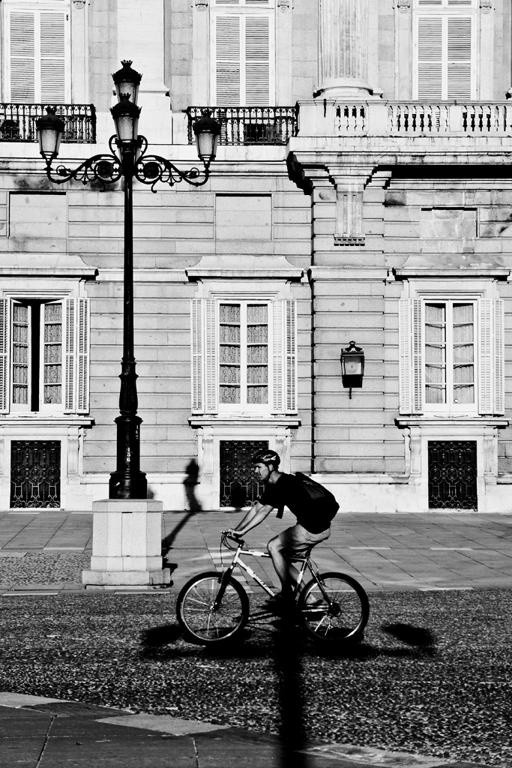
[221,449,332,615]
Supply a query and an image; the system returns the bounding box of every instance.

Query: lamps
[341,340,365,376]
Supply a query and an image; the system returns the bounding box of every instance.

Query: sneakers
[267,594,292,602]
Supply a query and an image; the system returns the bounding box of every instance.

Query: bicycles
[175,527,371,648]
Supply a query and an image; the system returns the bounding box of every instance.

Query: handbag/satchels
[295,471,339,521]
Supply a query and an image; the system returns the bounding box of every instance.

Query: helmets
[253,450,279,465]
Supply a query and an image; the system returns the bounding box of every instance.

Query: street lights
[29,57,222,500]
